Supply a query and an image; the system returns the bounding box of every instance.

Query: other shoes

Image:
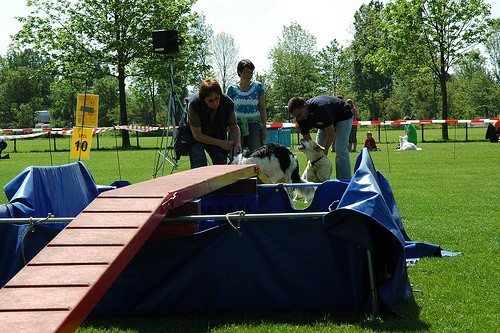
[351,149,357,152]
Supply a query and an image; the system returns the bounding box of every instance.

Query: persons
[287,96,353,182]
[346,99,358,153]
[404,116,417,145]
[226,59,267,161]
[364,131,377,151]
[174,79,242,169]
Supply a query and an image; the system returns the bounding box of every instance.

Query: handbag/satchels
[173,94,199,160]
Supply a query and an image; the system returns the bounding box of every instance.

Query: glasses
[294,111,302,119]
[205,96,220,103]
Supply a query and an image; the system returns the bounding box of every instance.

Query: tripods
[152,54,211,179]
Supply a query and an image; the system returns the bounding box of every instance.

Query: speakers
[152,29,182,54]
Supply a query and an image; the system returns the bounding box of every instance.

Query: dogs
[397,135,422,151]
[293,137,332,203]
[227,142,318,200]
